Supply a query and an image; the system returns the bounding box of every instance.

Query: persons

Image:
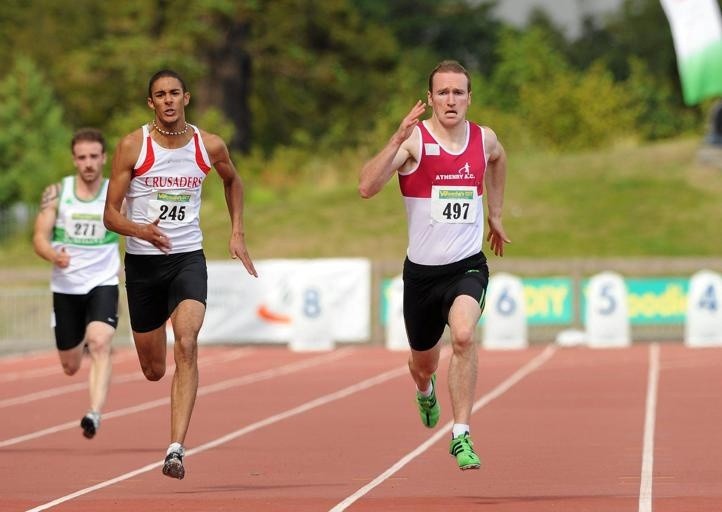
[33,126,127,438]
[103,69,258,480]
[359,60,512,470]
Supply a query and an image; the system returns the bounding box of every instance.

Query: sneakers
[81,413,98,438]
[449,431,480,469]
[416,373,440,429]
[162,452,185,480]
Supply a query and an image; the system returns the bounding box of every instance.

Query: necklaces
[152,119,188,135]
